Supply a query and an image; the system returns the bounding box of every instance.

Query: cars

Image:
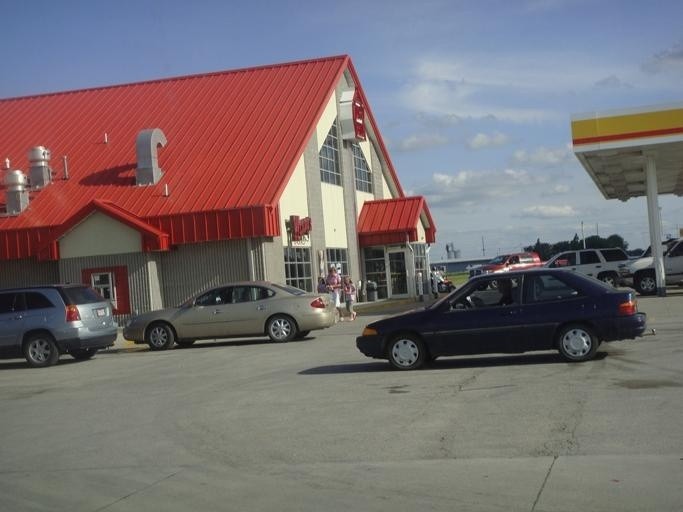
[122,279,340,351]
[2,285,118,368]
[355,265,648,371]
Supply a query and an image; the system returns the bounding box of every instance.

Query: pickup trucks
[467,251,567,280]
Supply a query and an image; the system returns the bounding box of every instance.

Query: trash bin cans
[366,279,378,301]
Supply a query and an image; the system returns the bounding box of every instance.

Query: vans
[619,238,682,295]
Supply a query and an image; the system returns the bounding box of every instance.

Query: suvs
[543,246,632,287]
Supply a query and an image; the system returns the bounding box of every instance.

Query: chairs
[228,289,266,301]
[510,287,520,304]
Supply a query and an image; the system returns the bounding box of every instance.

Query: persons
[324,266,344,321]
[316,277,326,293]
[342,275,356,321]
